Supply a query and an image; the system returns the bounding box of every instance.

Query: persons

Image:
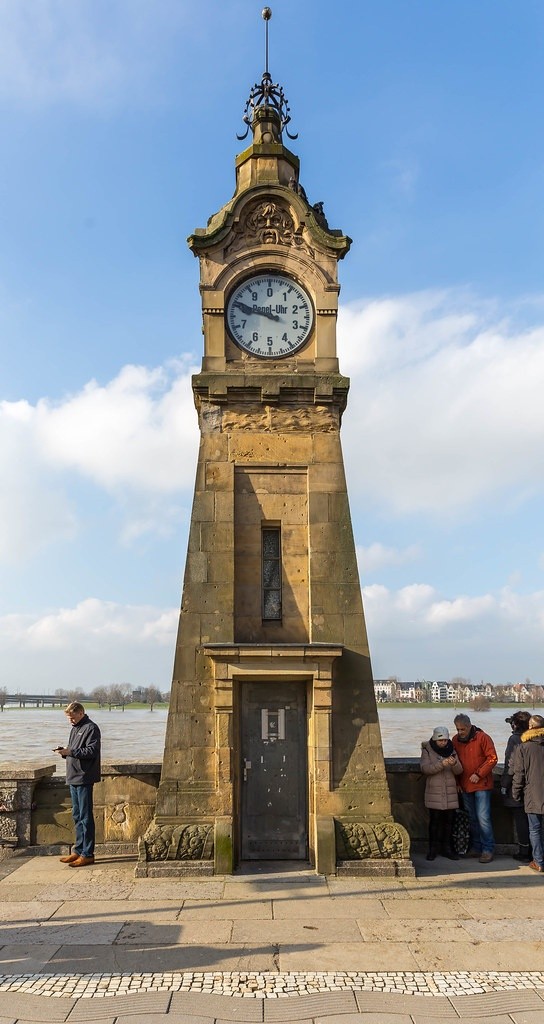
[450,714,498,864]
[511,714,544,873]
[52,702,101,867]
[500,710,533,864]
[419,727,464,862]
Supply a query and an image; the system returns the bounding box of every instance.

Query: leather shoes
[60,852,94,867]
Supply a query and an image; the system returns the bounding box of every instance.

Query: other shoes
[426,853,436,861]
[442,852,459,859]
[513,852,532,862]
[460,848,482,858]
[479,851,495,863]
[529,860,544,872]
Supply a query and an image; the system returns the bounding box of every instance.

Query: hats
[505,715,527,724]
[432,726,450,741]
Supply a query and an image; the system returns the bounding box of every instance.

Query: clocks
[224,270,315,360]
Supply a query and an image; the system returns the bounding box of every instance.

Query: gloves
[512,794,521,801]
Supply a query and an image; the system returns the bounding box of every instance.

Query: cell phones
[451,753,456,757]
